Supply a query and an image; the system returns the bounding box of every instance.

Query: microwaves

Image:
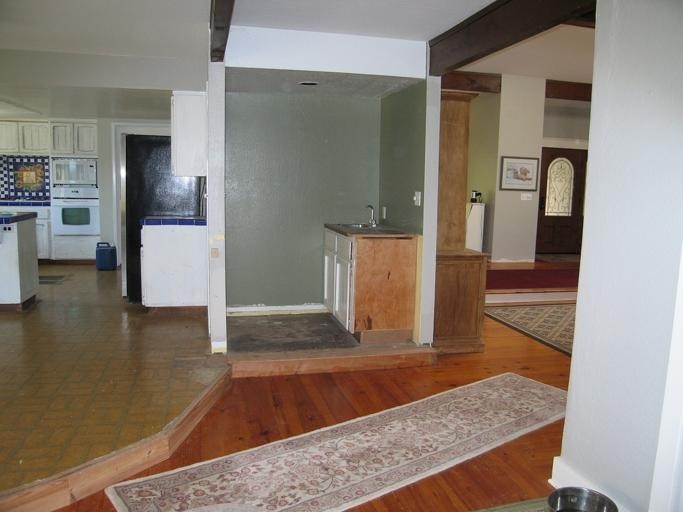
[51,158,97,187]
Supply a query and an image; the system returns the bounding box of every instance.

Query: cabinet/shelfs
[321,229,415,337]
[35,210,49,259]
[0,122,47,154]
[50,122,96,155]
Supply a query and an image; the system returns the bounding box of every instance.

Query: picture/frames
[498,156,540,192]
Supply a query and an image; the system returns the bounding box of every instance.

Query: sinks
[338,223,369,229]
[0,212,17,217]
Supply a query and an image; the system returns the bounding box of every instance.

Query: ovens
[49,197,101,236]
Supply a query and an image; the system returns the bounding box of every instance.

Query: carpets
[103,369,566,511]
[482,302,576,358]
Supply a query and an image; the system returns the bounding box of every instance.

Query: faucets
[364,204,377,228]
